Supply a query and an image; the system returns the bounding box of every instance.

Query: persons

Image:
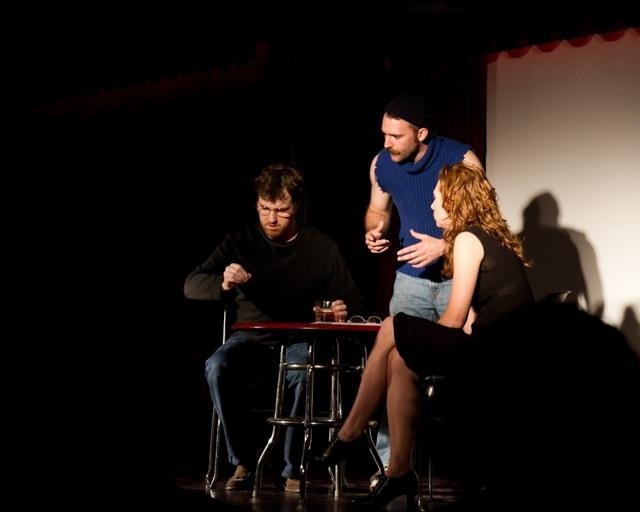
[312,159,536,510]
[364,95,486,489]
[183,163,364,492]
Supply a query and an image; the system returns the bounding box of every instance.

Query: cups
[316,300,339,324]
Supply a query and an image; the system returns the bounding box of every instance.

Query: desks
[229,321,387,505]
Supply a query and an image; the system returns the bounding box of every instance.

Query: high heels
[308,432,370,483]
[347,469,419,510]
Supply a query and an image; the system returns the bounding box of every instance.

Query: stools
[205,309,226,490]
[415,373,444,502]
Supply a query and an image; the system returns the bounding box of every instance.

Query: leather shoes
[283,477,312,493]
[225,463,254,490]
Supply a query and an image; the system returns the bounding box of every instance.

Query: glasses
[256,206,291,218]
